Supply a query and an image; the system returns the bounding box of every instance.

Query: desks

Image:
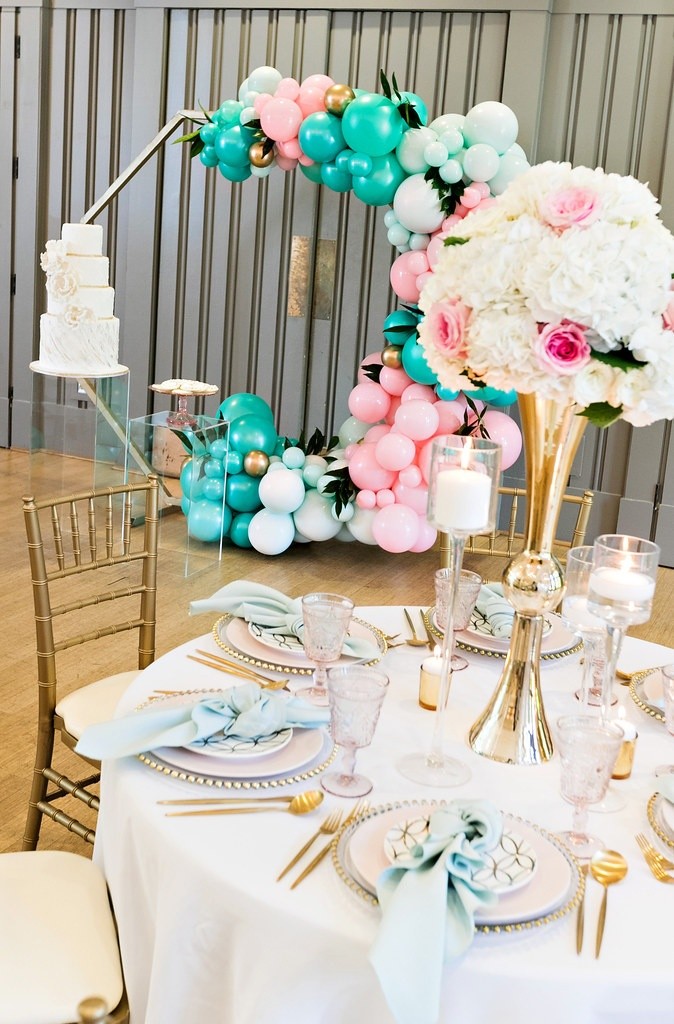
[92,606,674,1024]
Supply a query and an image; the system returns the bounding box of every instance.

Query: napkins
[473,584,520,637]
[362,801,502,1024]
[189,581,385,661]
[76,684,328,760]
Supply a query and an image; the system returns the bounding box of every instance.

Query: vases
[468,392,595,766]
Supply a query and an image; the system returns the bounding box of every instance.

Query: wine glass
[400,433,500,786]
[294,592,355,705]
[554,717,624,862]
[321,664,389,800]
[561,532,662,744]
[421,568,484,672]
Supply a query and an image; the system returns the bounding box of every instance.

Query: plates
[213,610,386,669]
[642,668,663,712]
[343,804,578,926]
[131,689,338,790]
[427,608,583,656]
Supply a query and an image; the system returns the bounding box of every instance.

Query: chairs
[21,474,165,854]
[438,489,594,613]
[0,850,131,1023]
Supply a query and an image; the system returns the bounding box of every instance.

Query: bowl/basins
[651,764,674,832]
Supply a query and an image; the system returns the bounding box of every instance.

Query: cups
[419,665,453,710]
[663,666,673,735]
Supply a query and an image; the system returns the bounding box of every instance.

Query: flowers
[417,161,674,430]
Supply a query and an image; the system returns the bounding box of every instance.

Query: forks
[276,798,372,889]
[633,834,674,884]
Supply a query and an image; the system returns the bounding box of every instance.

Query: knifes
[158,796,297,804]
[196,648,290,691]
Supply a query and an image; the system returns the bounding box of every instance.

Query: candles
[429,434,500,539]
[590,531,658,625]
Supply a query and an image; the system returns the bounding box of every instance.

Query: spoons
[150,789,324,818]
[403,605,428,646]
[591,848,629,956]
[188,655,289,692]
